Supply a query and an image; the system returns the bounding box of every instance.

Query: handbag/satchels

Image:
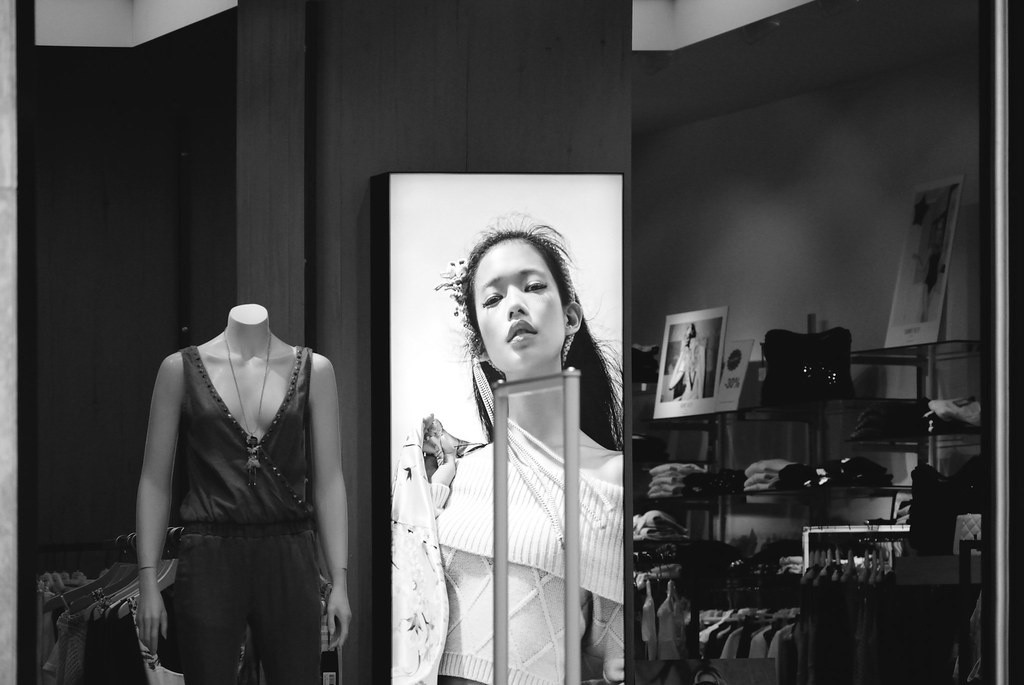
[952,513,982,556]
[755,327,856,403]
[907,453,987,552]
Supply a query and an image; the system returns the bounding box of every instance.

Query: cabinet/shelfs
[625,334,992,548]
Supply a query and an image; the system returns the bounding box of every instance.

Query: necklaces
[224,330,273,485]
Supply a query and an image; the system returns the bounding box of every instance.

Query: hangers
[34,523,187,623]
[621,522,987,633]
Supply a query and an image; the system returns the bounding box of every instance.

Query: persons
[392,210,626,685]
[914,217,948,324]
[669,323,699,401]
[137,302,353,684]
